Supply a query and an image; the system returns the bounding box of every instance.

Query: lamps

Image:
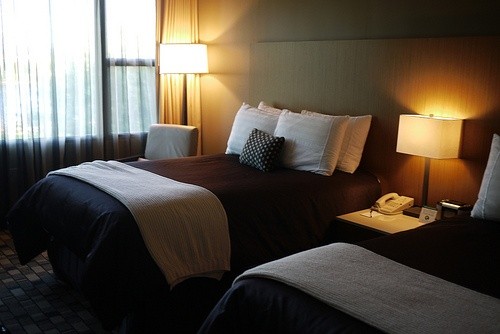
[395,114,464,218]
[159,43,208,127]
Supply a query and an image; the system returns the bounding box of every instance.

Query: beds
[197,211,500,334]
[8,152,378,334]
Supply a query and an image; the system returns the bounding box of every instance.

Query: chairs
[113,124,199,162]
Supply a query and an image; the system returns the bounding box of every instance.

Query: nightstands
[337,205,437,257]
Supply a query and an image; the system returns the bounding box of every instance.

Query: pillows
[470,134,500,220]
[256,101,282,116]
[225,102,280,154]
[238,128,284,172]
[275,109,350,176]
[302,110,372,173]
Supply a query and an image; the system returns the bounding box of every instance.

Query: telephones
[370,192,415,216]
